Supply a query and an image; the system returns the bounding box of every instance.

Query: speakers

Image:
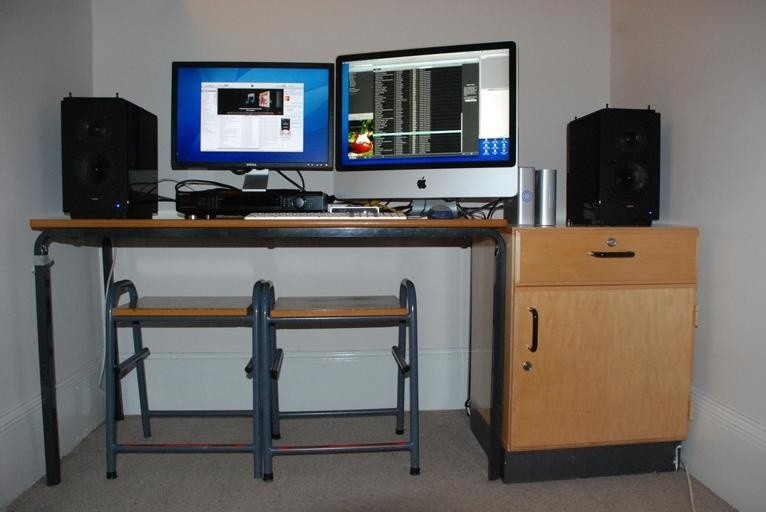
[61,97,157,218]
[566,107,661,226]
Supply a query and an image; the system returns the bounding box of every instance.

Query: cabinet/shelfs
[466,225,700,483]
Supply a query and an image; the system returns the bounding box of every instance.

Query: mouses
[428,205,452,219]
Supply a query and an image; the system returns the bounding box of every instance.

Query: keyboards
[244,212,408,221]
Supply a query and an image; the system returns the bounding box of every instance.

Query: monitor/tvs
[336,41,519,218]
[171,60,334,193]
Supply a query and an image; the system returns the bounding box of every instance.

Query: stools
[261,278,420,482]
[105,276,265,479]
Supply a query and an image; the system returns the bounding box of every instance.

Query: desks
[28,217,510,486]
